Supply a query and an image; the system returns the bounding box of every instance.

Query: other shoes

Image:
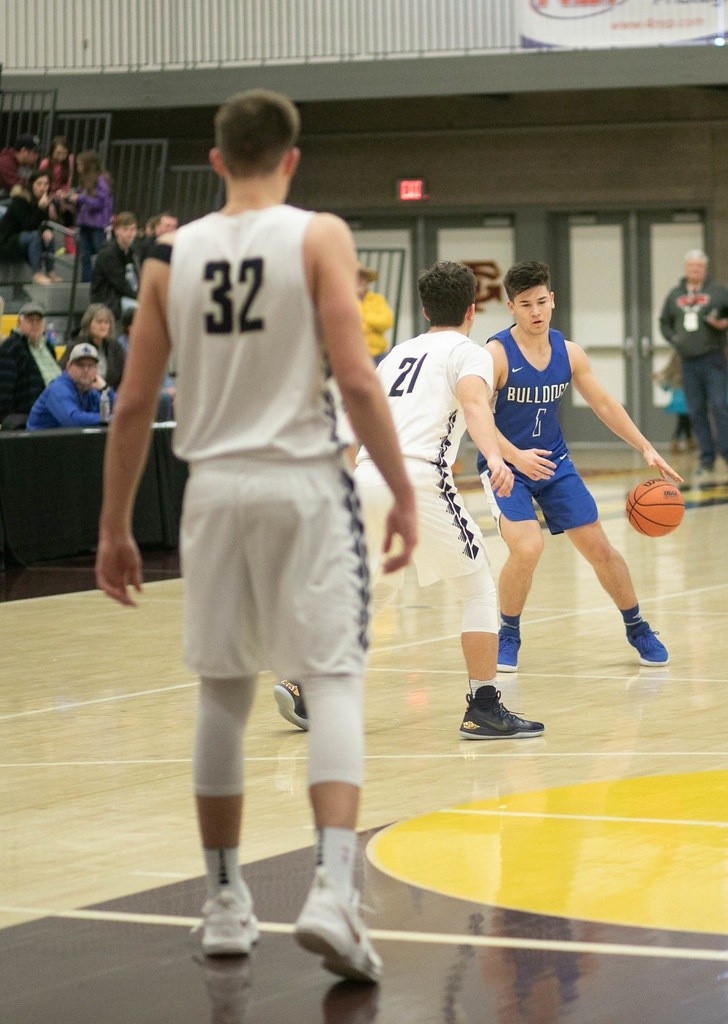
[687,440,696,450]
[669,442,679,454]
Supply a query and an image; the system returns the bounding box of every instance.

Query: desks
[1,419,180,568]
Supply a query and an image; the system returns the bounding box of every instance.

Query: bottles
[99,390,110,423]
[46,321,57,346]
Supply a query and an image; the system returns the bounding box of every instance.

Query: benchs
[0,186,177,391]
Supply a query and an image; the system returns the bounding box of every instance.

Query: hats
[68,343,101,364]
[19,303,45,317]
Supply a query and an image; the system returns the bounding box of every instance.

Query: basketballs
[626,479,685,537]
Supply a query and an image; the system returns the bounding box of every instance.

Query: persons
[27,347,115,429]
[96,91,417,983]
[355,264,397,366]
[658,250,728,475]
[0,305,62,431]
[55,149,112,283]
[39,135,74,194]
[0,132,62,283]
[476,265,684,673]
[90,210,142,315]
[274,260,545,739]
[56,303,170,423]
[141,210,178,261]
[653,349,694,452]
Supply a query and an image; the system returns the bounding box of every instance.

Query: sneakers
[459,685,545,740]
[496,626,522,672]
[626,621,669,666]
[190,879,259,955]
[273,679,308,730]
[291,867,381,982]
